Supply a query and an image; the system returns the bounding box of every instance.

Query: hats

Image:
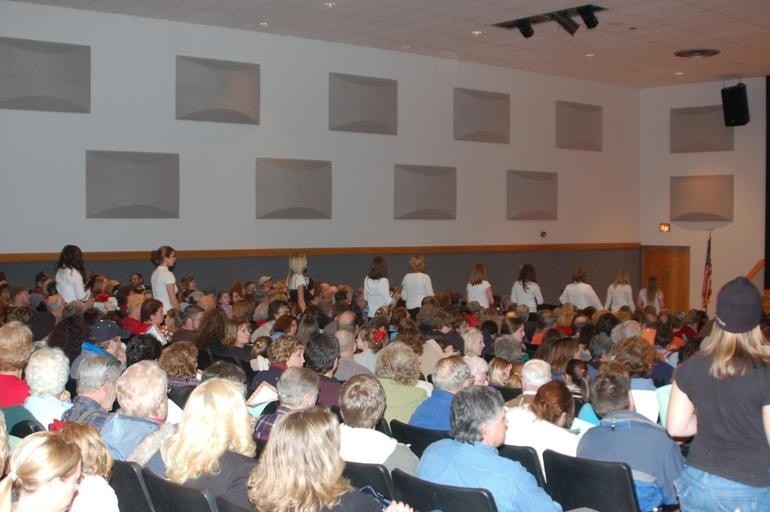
[714,276,763,333]
[28,311,56,341]
[86,319,131,344]
[259,276,274,286]
[7,283,31,299]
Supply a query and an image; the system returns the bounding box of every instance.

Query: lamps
[490,4,611,37]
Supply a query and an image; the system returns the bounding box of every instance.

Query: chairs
[490,385,522,403]
[216,495,251,512]
[8,420,43,439]
[389,418,452,459]
[522,342,540,359]
[392,468,499,512]
[196,347,258,386]
[329,405,389,435]
[544,451,641,512]
[342,462,400,501]
[574,398,584,418]
[143,467,212,511]
[110,461,155,512]
[498,446,548,495]
[168,386,195,410]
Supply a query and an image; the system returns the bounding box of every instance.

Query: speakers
[720,82,751,127]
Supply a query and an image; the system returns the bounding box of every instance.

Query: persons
[0,247,709,512]
[666,277,770,512]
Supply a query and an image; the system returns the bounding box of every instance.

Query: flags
[701,239,712,310]
